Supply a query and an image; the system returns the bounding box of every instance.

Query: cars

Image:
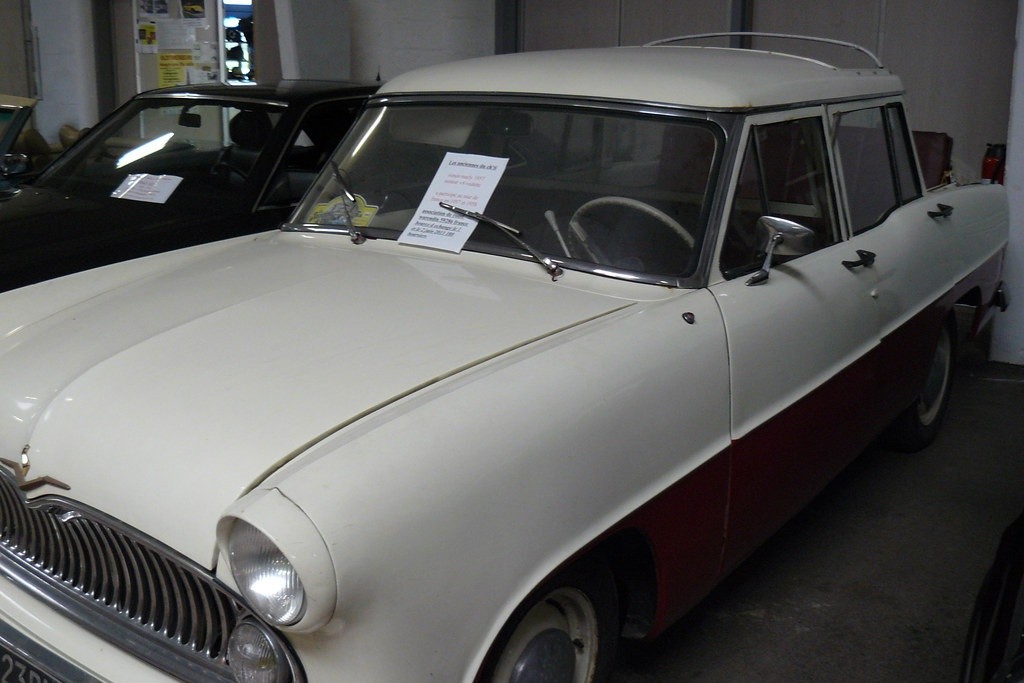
[0,31,1013,683]
[0,75,385,294]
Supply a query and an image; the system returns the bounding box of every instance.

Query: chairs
[58,123,104,174]
[210,109,271,193]
[10,128,51,168]
